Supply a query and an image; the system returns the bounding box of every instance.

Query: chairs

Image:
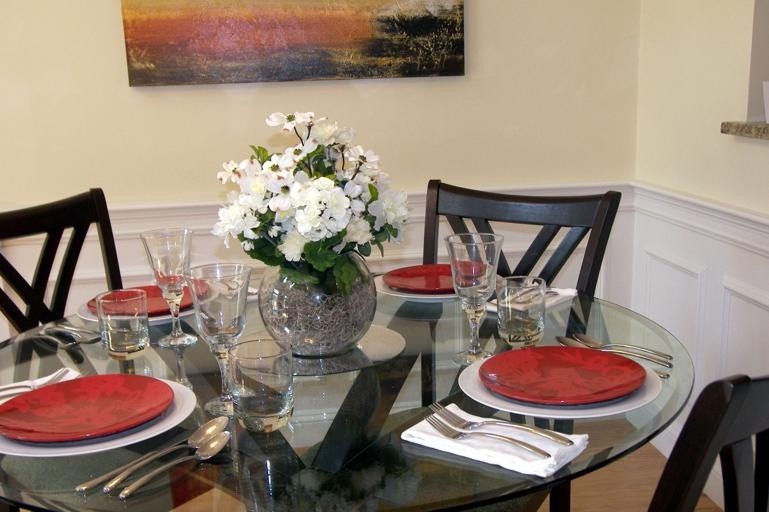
[648,375,769,511]
[422,178,621,512]
[0,189,135,384]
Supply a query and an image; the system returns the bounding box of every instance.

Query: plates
[1,379,196,458]
[382,264,453,293]
[479,345,647,406]
[86,285,194,317]
[1,374,174,444]
[76,281,220,327]
[458,354,664,419]
[373,273,505,304]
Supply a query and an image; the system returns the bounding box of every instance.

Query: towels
[486,283,578,312]
[400,403,591,479]
[0,368,81,405]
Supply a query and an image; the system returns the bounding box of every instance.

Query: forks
[1,367,70,392]
[423,399,574,459]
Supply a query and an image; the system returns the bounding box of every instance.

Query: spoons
[554,332,673,369]
[104,417,231,502]
[38,327,103,349]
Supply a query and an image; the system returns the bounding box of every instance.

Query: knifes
[57,322,101,335]
[75,426,199,493]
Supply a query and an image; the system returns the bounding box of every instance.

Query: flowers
[210,112,408,297]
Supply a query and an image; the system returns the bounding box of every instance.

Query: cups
[496,276,546,346]
[228,338,293,434]
[96,289,150,361]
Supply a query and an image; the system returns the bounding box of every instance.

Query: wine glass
[445,232,505,364]
[183,264,254,417]
[139,228,198,347]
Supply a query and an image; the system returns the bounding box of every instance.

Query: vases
[259,251,377,359]
